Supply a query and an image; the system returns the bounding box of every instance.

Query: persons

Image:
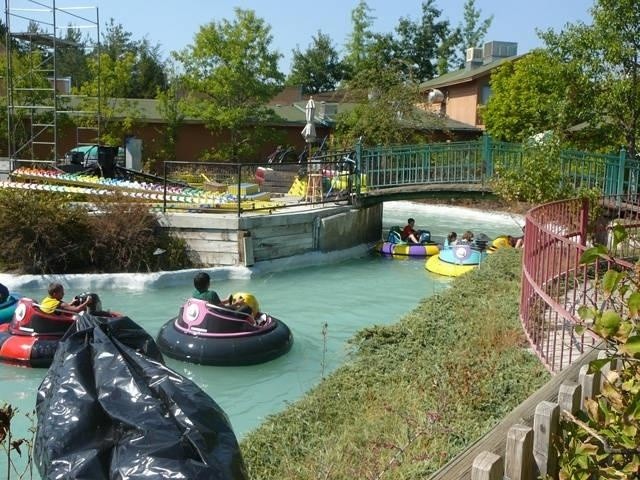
[514,225,525,248]
[40,281,92,316]
[461,231,474,244]
[401,218,420,243]
[446,231,462,248]
[508,235,515,247]
[191,272,267,327]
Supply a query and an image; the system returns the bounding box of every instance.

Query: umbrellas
[301,95,317,155]
